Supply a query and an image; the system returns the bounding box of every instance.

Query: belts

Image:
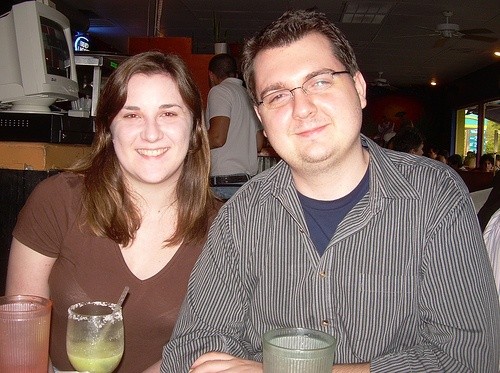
[209,176,252,185]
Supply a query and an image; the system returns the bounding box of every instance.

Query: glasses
[257,71,351,110]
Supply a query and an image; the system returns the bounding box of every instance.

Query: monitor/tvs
[0,0,80,115]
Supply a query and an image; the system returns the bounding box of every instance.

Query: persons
[373,118,499,173]
[5,51,223,373]
[160,10,499,373]
[204,54,265,204]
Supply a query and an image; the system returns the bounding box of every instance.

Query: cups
[66,301,124,373]
[0,295,52,373]
[263,328,336,373]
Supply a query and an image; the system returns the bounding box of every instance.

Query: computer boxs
[0,113,94,144]
